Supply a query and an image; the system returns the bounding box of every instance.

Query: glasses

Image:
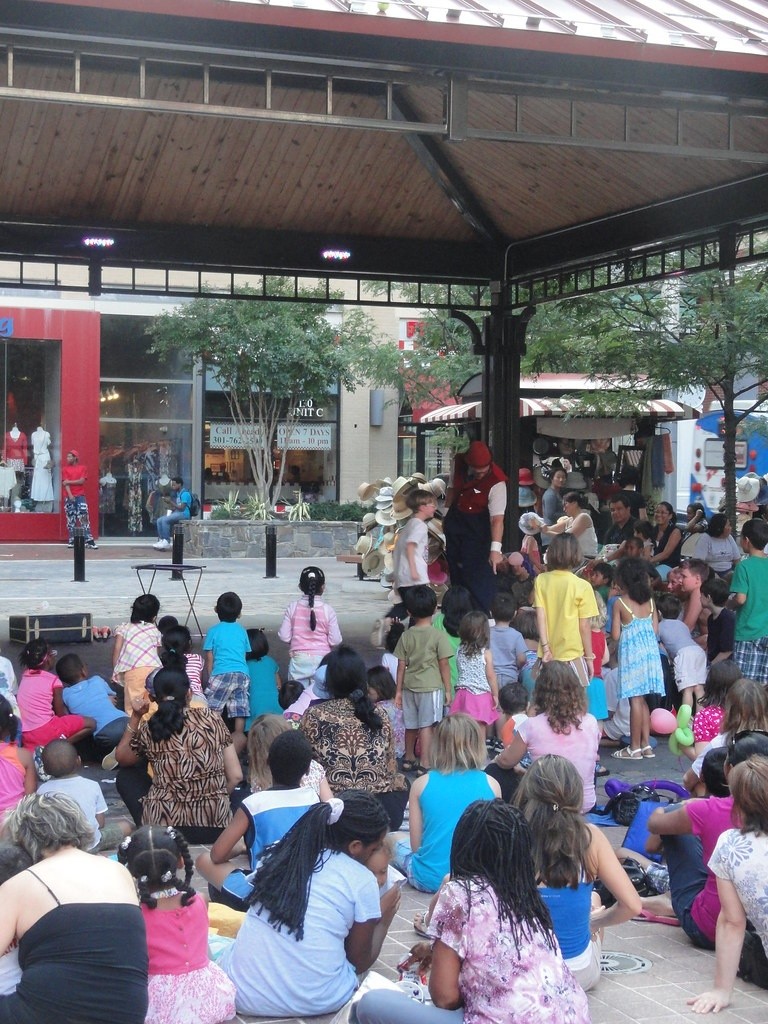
[425,502,436,509]
[49,650,57,658]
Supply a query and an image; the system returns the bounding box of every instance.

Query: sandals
[642,745,655,758]
[401,758,431,778]
[610,745,644,760]
[596,765,610,776]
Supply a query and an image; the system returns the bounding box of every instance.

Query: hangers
[635,421,671,439]
[102,438,177,460]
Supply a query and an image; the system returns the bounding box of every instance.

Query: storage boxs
[8,612,93,645]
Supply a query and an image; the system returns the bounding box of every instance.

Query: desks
[132,563,206,638]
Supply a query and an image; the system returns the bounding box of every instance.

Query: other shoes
[369,619,385,647]
[153,540,162,548]
[101,746,119,771]
[158,539,173,549]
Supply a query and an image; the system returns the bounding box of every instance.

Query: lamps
[370,389,385,425]
[101,386,119,402]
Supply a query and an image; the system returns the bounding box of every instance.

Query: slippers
[413,912,432,939]
[631,909,681,926]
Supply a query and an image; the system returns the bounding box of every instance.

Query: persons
[0,426,28,499]
[153,475,174,518]
[0,565,641,1024]
[61,450,99,549]
[152,476,192,548]
[290,465,308,492]
[370,489,437,648]
[30,426,55,502]
[216,462,230,481]
[100,472,117,514]
[492,460,768,1014]
[441,441,507,595]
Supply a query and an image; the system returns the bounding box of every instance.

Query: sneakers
[486,736,505,753]
[85,540,99,549]
[68,542,75,547]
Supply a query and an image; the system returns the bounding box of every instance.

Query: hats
[532,435,614,489]
[518,487,537,508]
[464,441,494,467]
[716,471,768,513]
[518,511,542,535]
[519,468,536,485]
[70,450,80,462]
[355,472,450,605]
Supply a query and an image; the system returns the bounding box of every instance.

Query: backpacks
[180,490,201,516]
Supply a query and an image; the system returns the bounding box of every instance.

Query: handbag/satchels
[593,855,649,909]
